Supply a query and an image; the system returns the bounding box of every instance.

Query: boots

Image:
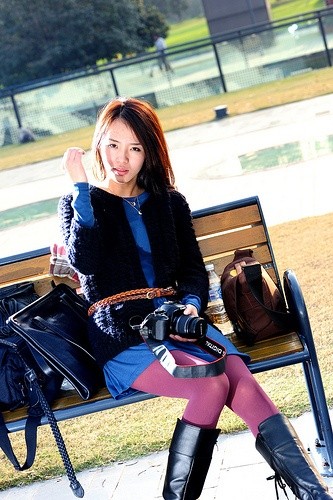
[161,419,222,500]
[255,413,333,500]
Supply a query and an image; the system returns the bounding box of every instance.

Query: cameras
[146,301,207,341]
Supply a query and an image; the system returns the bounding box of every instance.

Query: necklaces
[110,189,141,215]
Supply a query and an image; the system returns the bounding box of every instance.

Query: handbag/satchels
[6,281,104,401]
[220,248,289,344]
[0,284,84,499]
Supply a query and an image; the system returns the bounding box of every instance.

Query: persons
[58,98,333,500]
[154,33,175,75]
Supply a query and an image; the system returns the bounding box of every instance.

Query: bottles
[205,263,237,344]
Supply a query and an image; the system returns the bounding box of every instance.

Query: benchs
[1,196,333,476]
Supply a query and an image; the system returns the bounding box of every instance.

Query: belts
[88,285,176,317]
